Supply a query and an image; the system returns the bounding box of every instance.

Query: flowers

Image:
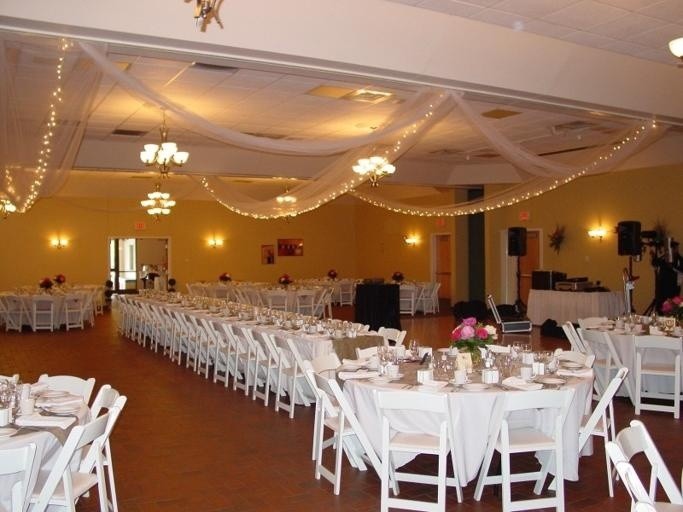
[54,274,65,284]
[40,277,53,290]
[451,317,494,351]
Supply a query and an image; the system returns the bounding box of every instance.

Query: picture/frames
[277,239,303,257]
[261,245,275,265]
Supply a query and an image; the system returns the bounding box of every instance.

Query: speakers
[507,227,527,256]
[618,221,641,255]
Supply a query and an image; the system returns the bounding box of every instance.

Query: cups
[383,364,399,377]
[416,369,432,383]
[10,379,25,413]
[281,320,291,330]
[208,306,217,312]
[519,367,534,379]
[483,367,497,387]
[453,369,468,382]
[327,323,335,339]
[408,338,418,356]
[543,350,553,368]
[238,312,248,320]
[180,301,188,307]
[532,351,543,368]
[511,341,521,364]
[443,359,458,389]
[306,324,315,334]
[520,344,531,364]
[375,339,386,358]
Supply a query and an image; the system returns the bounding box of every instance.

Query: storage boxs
[487,294,533,334]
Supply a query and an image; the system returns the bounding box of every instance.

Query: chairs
[6,294,31,333]
[400,284,414,317]
[294,294,315,317]
[0,375,19,384]
[76,286,97,328]
[243,290,264,308]
[79,385,126,512]
[146,303,170,352]
[226,324,264,396]
[563,324,585,354]
[376,389,466,511]
[430,283,440,313]
[159,306,187,359]
[214,287,229,301]
[168,310,194,366]
[604,417,683,512]
[339,284,353,307]
[232,289,246,304]
[1,443,37,512]
[0,297,7,333]
[61,294,84,331]
[179,312,213,372]
[135,300,156,348]
[577,330,633,408]
[246,328,313,407]
[266,293,288,312]
[94,285,104,317]
[190,315,235,379]
[32,296,55,333]
[311,367,391,497]
[38,372,95,408]
[547,369,629,497]
[268,334,318,419]
[633,337,682,419]
[353,345,405,362]
[577,317,608,326]
[207,320,244,387]
[475,388,577,512]
[37,414,112,511]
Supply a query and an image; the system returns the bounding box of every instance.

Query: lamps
[415,283,441,315]
[667,36,682,63]
[140,184,177,224]
[351,156,396,190]
[0,191,17,221]
[139,120,189,180]
[276,196,298,218]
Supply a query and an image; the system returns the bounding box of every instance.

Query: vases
[463,346,482,367]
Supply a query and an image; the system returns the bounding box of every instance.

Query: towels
[192,309,209,313]
[501,376,544,392]
[555,364,594,381]
[13,411,77,432]
[336,370,378,381]
[417,379,448,394]
[216,316,240,321]
[252,324,276,330]
[165,304,181,307]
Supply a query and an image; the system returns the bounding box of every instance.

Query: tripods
[512,256,527,316]
[623,255,635,314]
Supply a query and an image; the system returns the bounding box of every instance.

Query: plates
[449,378,473,386]
[563,361,583,369]
[538,376,565,386]
[40,394,86,416]
[0,427,18,437]
